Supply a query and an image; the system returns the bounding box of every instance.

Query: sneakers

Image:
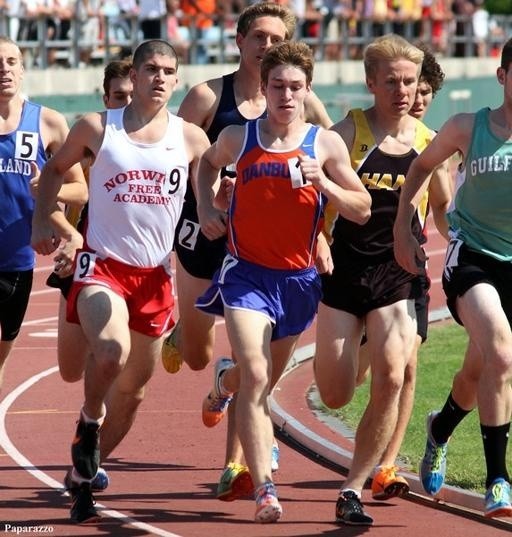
[334,488,374,525]
[215,462,255,503]
[483,477,512,518]
[271,438,280,472]
[252,480,282,523]
[64,469,102,525]
[90,468,110,493]
[419,410,453,497]
[160,329,185,375]
[201,358,235,429]
[70,400,107,481]
[371,463,410,501]
